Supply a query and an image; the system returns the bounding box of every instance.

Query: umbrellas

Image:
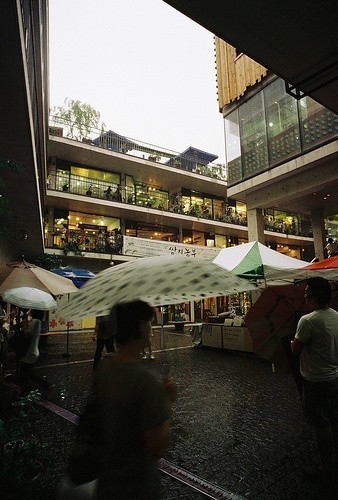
[1,287,57,310]
[244,280,338,364]
[50,266,96,278]
[0,255,79,310]
[56,254,258,323]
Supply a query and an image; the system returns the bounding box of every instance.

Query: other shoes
[305,462,332,475]
[142,355,156,360]
[42,381,53,400]
[9,395,31,406]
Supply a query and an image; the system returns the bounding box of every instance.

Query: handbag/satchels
[65,364,107,485]
[292,351,304,401]
[8,329,30,359]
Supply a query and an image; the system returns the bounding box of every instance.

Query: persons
[105,186,123,201]
[327,238,338,258]
[97,230,115,246]
[61,224,67,243]
[202,205,209,217]
[79,228,85,250]
[68,300,178,500]
[0,306,25,378]
[289,277,338,477]
[9,308,55,408]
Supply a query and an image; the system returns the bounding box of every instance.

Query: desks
[201,324,255,355]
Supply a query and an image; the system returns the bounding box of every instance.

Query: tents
[208,241,313,278]
